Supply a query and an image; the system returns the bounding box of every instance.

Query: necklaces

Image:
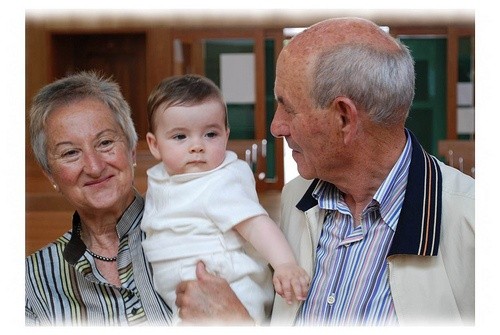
[75,225,118,263]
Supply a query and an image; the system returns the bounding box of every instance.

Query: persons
[139,73,310,322]
[25,73,256,321]
[267,17,475,322]
